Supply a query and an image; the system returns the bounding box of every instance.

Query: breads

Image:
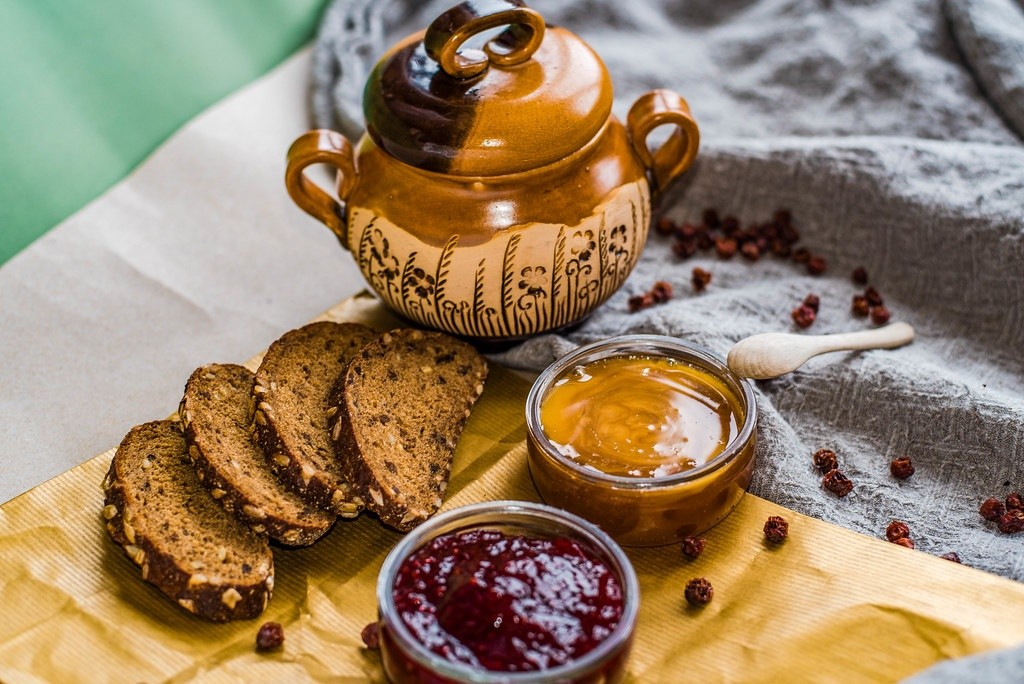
[103,418,274,622]
[248,321,387,520]
[327,328,489,534]
[178,362,335,549]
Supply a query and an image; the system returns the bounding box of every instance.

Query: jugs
[284,0,699,352]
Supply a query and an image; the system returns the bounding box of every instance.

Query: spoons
[727,320,915,381]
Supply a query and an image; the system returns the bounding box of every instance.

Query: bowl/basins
[524,337,757,544]
[376,501,639,684]
